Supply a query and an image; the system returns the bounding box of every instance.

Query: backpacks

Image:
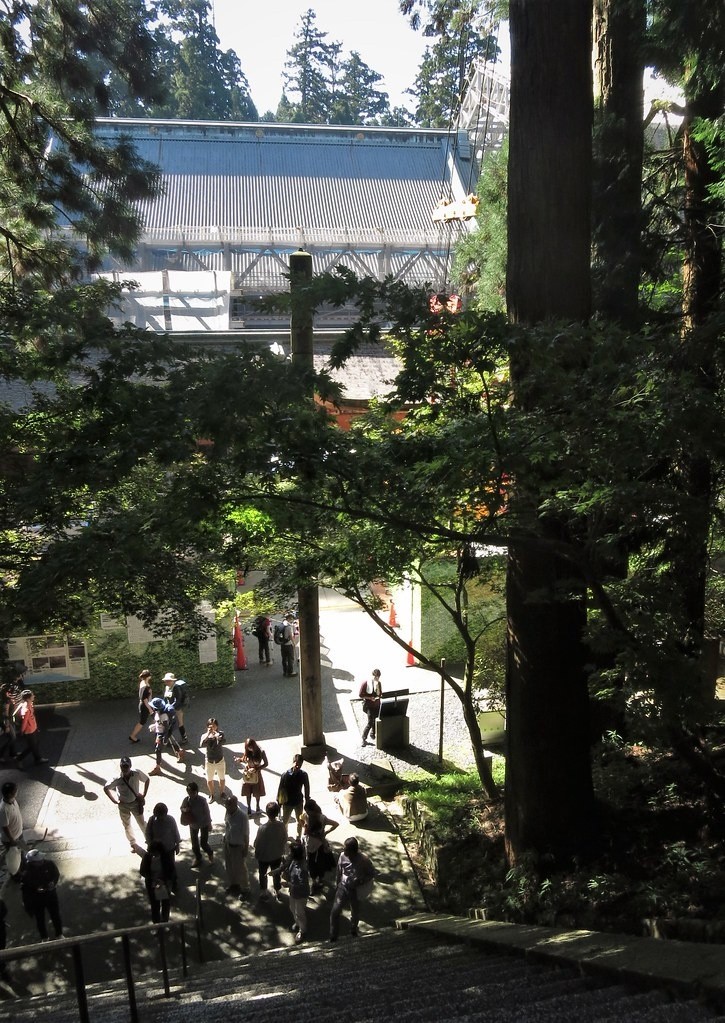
[273,624,291,644]
[172,681,189,711]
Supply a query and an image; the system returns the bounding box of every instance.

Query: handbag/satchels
[356,878,375,901]
[155,884,169,900]
[244,766,258,784]
[278,773,288,805]
[138,799,144,815]
[181,797,193,825]
[283,859,293,881]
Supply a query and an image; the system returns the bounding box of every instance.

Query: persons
[0,683,49,772]
[277,754,310,843]
[199,717,228,804]
[139,782,375,944]
[334,771,369,822]
[128,670,189,776]
[103,756,150,853]
[0,783,65,950]
[252,611,300,677]
[359,669,382,748]
[233,737,268,818]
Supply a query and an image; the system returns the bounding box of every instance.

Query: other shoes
[192,857,202,868]
[209,797,216,804]
[14,757,23,769]
[261,892,268,900]
[149,767,161,776]
[219,794,225,804]
[274,892,281,903]
[352,928,358,938]
[238,889,250,902]
[209,851,213,860]
[295,933,307,942]
[35,758,49,764]
[327,937,335,943]
[289,672,298,676]
[291,922,299,931]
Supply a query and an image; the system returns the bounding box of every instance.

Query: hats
[148,698,165,709]
[162,672,176,680]
[26,849,48,863]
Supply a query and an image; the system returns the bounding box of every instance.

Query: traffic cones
[387,603,400,628]
[405,640,419,667]
